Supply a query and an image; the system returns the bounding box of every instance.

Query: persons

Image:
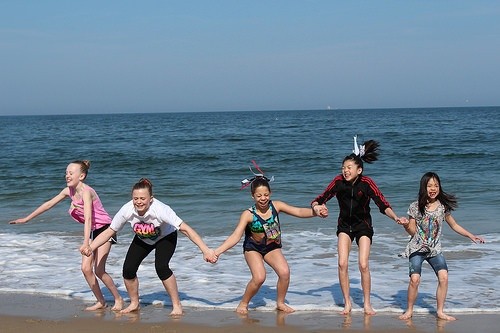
[203,176,328,314]
[80,178,218,316]
[9,160,125,311]
[310,139,410,315]
[399,172,485,320]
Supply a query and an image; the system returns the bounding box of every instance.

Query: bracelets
[312,204,319,209]
